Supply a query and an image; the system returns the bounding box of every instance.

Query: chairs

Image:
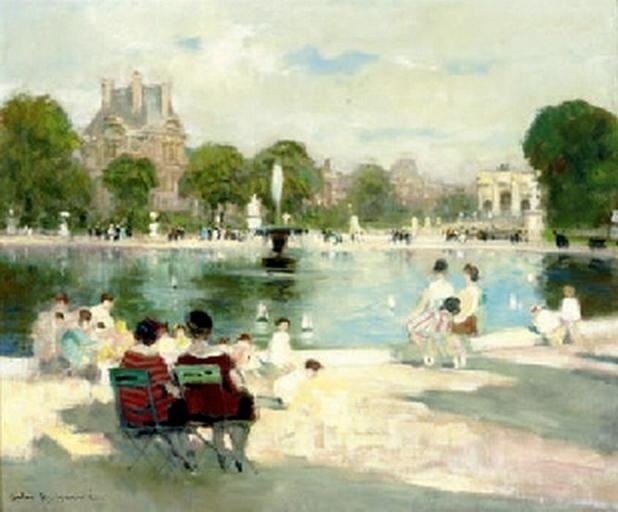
[165,364,255,477]
[108,368,193,485]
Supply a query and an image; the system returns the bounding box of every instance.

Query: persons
[558,284,583,341]
[81,216,575,247]
[404,258,456,330]
[28,281,324,476]
[449,260,483,337]
[529,303,568,347]
[408,296,464,363]
